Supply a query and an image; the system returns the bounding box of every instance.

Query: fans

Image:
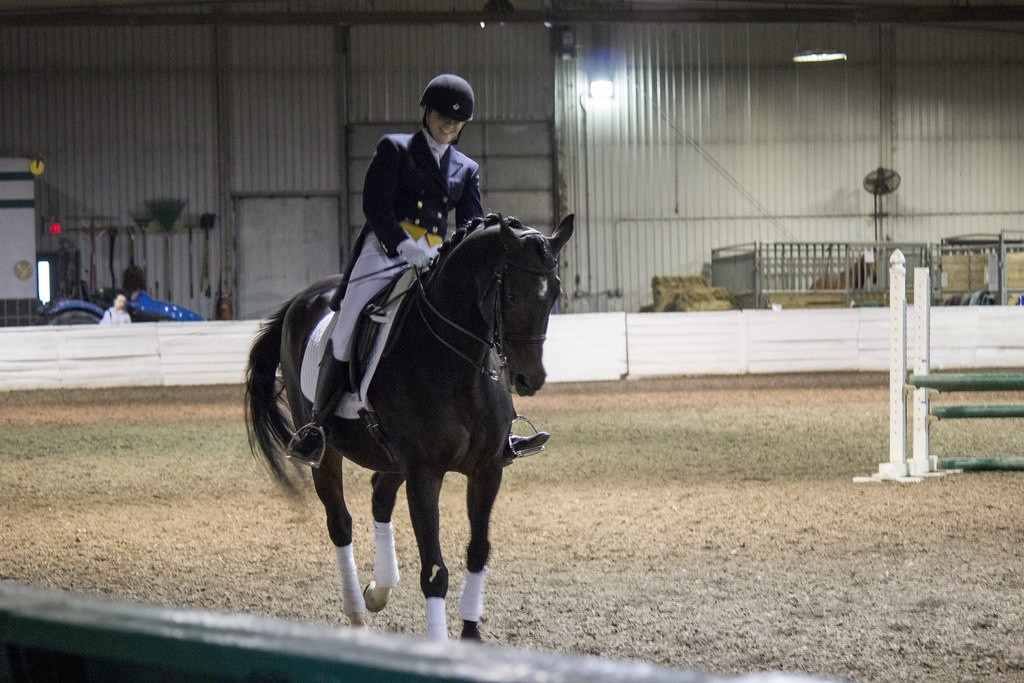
[862,167,902,197]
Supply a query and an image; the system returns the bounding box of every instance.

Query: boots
[288,338,349,461]
[501,431,550,467]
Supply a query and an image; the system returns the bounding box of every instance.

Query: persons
[286,76,550,463]
[98,290,131,324]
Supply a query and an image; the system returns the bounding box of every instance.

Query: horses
[244,215,574,642]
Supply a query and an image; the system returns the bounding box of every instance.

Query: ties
[432,149,441,168]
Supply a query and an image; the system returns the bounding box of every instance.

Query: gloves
[401,242,430,269]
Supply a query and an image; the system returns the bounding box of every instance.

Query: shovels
[200,212,218,296]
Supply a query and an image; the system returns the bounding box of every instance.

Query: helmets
[419,74,474,122]
